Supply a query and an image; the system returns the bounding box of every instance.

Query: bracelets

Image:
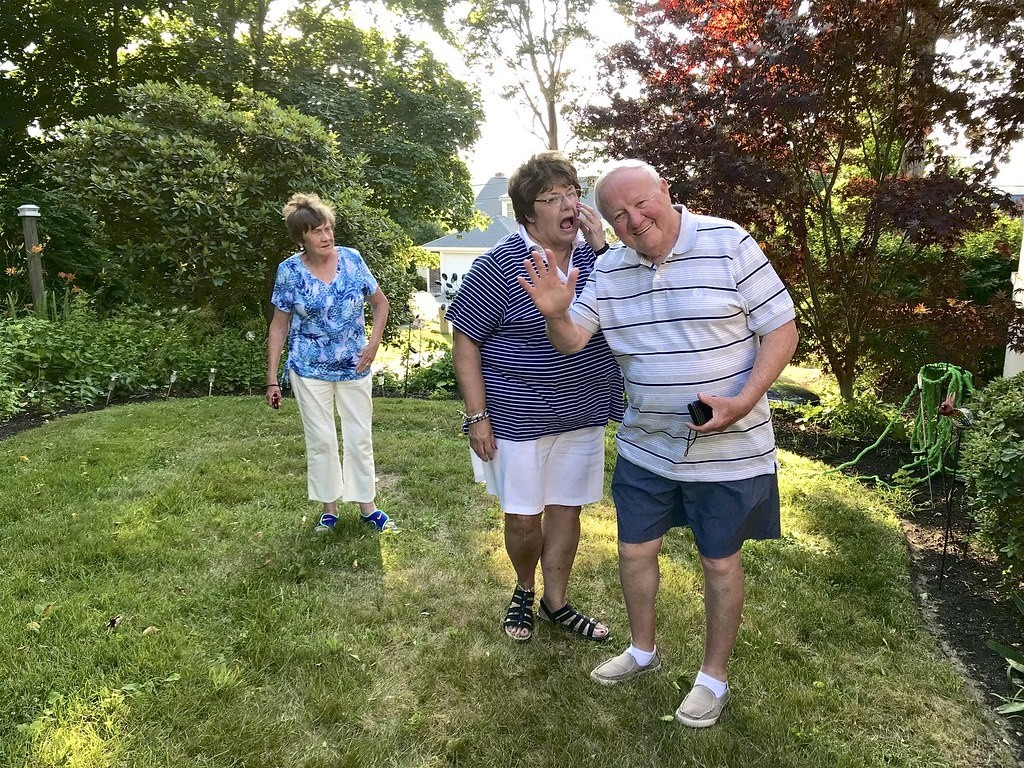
[594,241,609,256]
[267,383,279,388]
[465,408,490,425]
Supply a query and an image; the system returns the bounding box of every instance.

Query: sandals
[536,598,609,641]
[359,510,398,532]
[503,584,535,643]
[315,512,339,533]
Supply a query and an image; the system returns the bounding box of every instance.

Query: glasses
[534,188,582,207]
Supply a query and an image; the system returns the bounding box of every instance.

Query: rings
[366,363,369,366]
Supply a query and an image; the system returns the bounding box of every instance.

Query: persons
[444,151,626,641]
[265,192,398,535]
[519,157,799,727]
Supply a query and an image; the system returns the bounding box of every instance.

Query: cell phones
[687,400,712,426]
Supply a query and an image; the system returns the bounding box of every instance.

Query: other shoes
[676,682,731,727]
[591,645,661,686]
